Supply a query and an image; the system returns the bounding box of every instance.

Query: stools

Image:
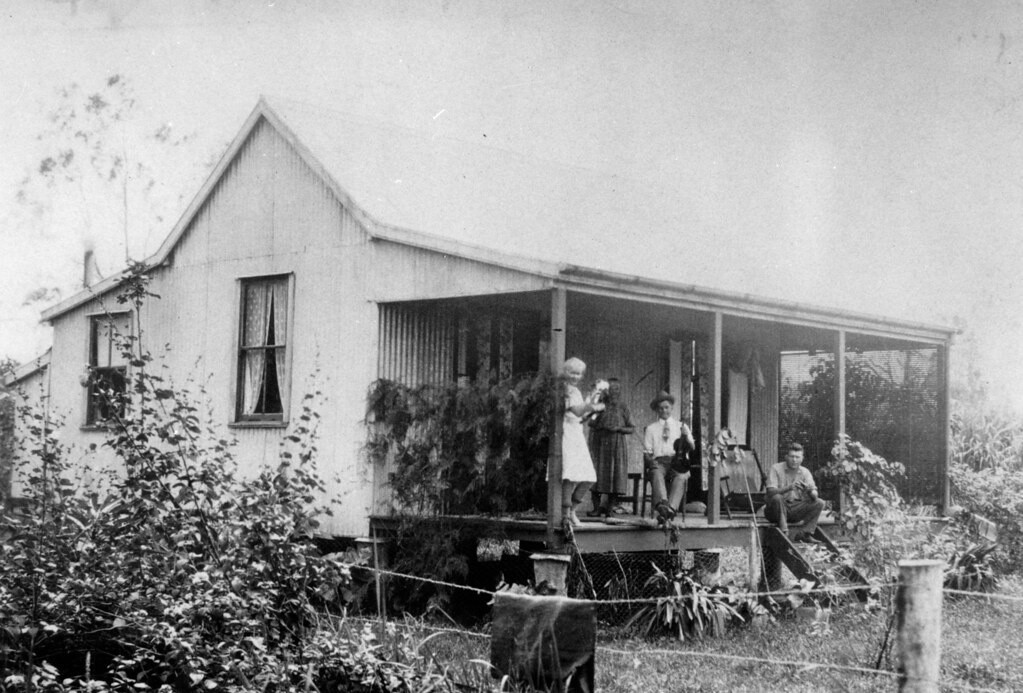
[593,472,641,515]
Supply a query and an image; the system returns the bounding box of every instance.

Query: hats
[650,389,675,411]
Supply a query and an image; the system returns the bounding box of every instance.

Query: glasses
[662,426,669,442]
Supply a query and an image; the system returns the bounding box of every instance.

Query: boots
[587,492,602,517]
[606,494,618,517]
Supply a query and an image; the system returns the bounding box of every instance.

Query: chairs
[641,427,690,521]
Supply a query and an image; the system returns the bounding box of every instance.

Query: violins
[671,416,691,473]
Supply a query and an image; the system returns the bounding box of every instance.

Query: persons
[642,390,695,524]
[763,443,824,544]
[587,378,636,516]
[545,356,609,527]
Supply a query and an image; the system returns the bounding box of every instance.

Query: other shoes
[795,532,821,543]
[564,511,581,526]
[657,510,674,524]
[657,504,669,515]
